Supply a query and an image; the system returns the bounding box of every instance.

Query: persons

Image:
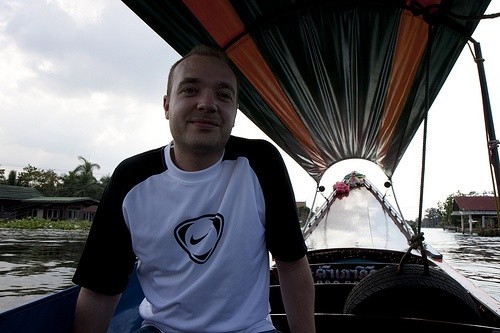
[69,43,316,333]
